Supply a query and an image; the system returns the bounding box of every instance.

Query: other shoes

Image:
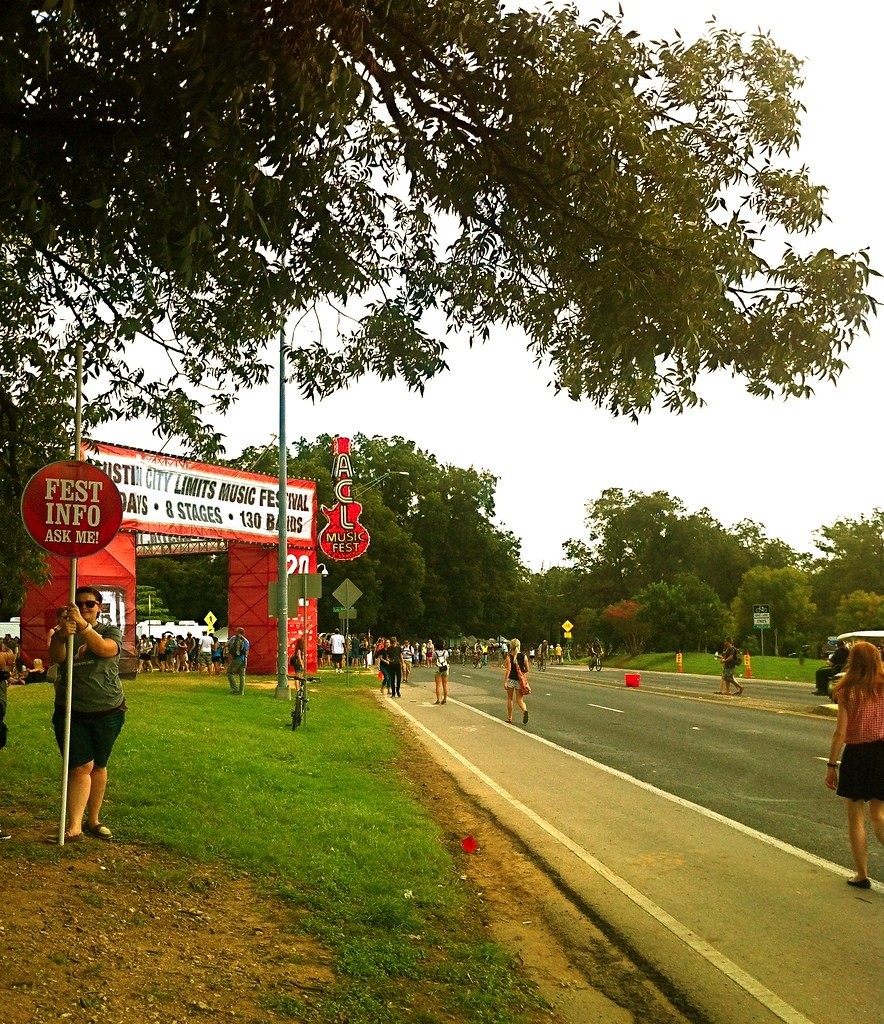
[380,688,385,695]
[388,687,391,693]
[335,671,338,673]
[340,671,344,674]
[397,691,401,698]
[391,695,395,699]
[136,667,221,677]
[82,821,113,839]
[811,689,829,695]
[0,829,11,840]
[435,700,440,704]
[847,877,871,888]
[715,685,746,696]
[47,832,84,843]
[441,700,446,705]
[238,691,244,696]
[505,719,511,722]
[523,710,529,724]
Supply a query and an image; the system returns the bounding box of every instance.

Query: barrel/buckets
[624,674,640,687]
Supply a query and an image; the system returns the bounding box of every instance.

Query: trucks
[0,617,21,639]
[136,620,209,640]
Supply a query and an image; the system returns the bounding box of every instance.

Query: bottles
[714,652,718,660]
[722,658,724,663]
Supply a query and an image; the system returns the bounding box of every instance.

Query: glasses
[75,600,100,608]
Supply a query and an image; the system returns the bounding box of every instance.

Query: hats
[167,635,172,638]
[390,637,396,642]
[187,632,192,635]
[234,627,245,635]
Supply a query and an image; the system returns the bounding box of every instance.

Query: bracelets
[827,762,838,767]
[80,622,92,633]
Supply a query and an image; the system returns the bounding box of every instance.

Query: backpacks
[434,651,449,673]
[228,635,244,656]
[731,647,742,666]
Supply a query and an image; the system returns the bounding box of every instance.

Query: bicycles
[285,675,323,731]
[589,653,603,671]
[473,651,483,669]
[538,655,548,672]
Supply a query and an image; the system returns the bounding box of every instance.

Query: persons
[294,639,305,691]
[444,638,510,666]
[317,629,437,698]
[591,638,603,663]
[47,587,127,842]
[719,637,744,695]
[504,639,529,724]
[530,640,563,669]
[0,634,47,724]
[432,640,449,704]
[225,628,249,696]
[46,606,68,686]
[811,641,849,694]
[825,642,884,888]
[135,631,225,676]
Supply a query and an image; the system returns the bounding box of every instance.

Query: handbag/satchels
[290,656,298,666]
[519,674,531,696]
